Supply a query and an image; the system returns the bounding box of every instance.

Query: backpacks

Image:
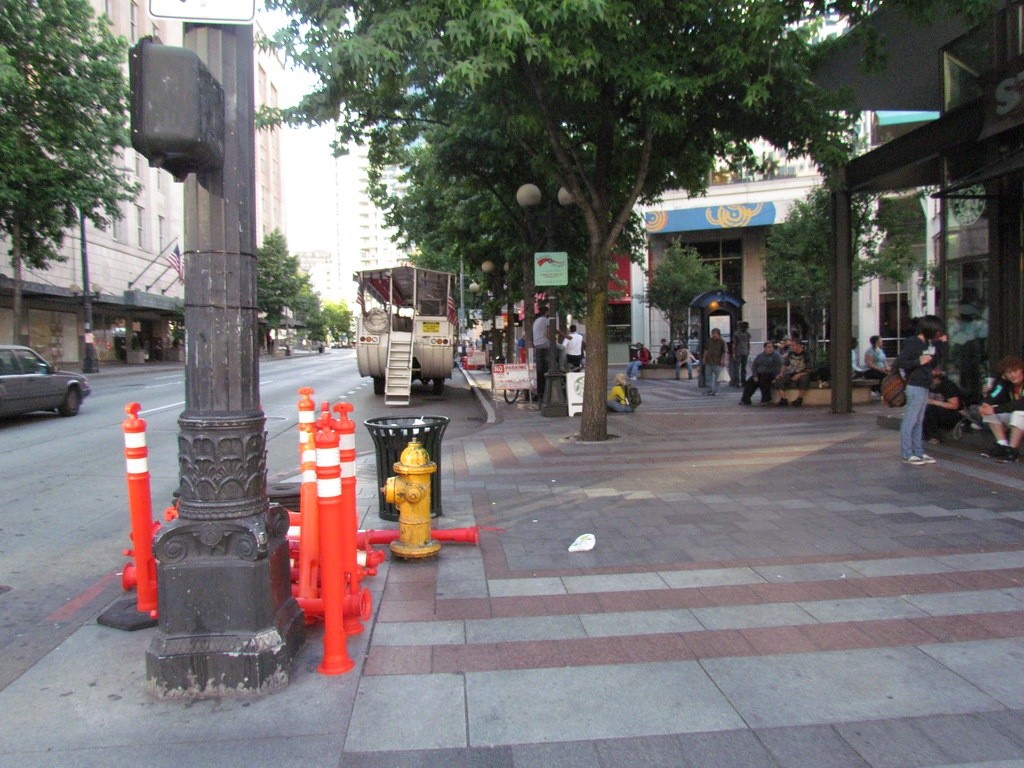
[617,384,642,408]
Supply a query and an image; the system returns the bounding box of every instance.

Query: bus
[354,265,457,407]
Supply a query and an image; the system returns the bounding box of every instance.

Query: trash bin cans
[363,415,450,521]
[629,344,639,362]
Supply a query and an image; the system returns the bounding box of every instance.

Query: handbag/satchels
[881,369,907,408]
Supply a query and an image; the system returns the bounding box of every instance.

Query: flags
[167,244,184,285]
[447,284,458,326]
[356,279,363,305]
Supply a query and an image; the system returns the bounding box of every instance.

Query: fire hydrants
[380,437,442,559]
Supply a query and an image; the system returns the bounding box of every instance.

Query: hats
[930,369,940,378]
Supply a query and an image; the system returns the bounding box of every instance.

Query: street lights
[515,181,578,417]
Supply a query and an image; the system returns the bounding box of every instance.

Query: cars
[0,344,92,417]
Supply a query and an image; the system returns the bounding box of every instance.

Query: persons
[738,341,783,405]
[774,338,812,407]
[979,355,1024,463]
[470,335,482,350]
[850,337,863,379]
[893,316,947,466]
[660,339,700,381]
[119,340,163,364]
[863,335,891,401]
[607,374,632,412]
[733,321,751,384]
[562,325,585,367]
[922,374,964,445]
[533,307,573,369]
[702,328,727,396]
[626,343,649,380]
[775,335,792,354]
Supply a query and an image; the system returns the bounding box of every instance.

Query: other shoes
[1001,446,1019,463]
[776,397,788,406]
[739,400,750,404]
[790,397,803,406]
[903,454,925,465]
[929,438,940,444]
[920,453,936,464]
[981,442,1008,458]
[761,400,769,405]
[869,390,880,401]
[630,375,637,380]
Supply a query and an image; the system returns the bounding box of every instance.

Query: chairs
[10,357,26,371]
